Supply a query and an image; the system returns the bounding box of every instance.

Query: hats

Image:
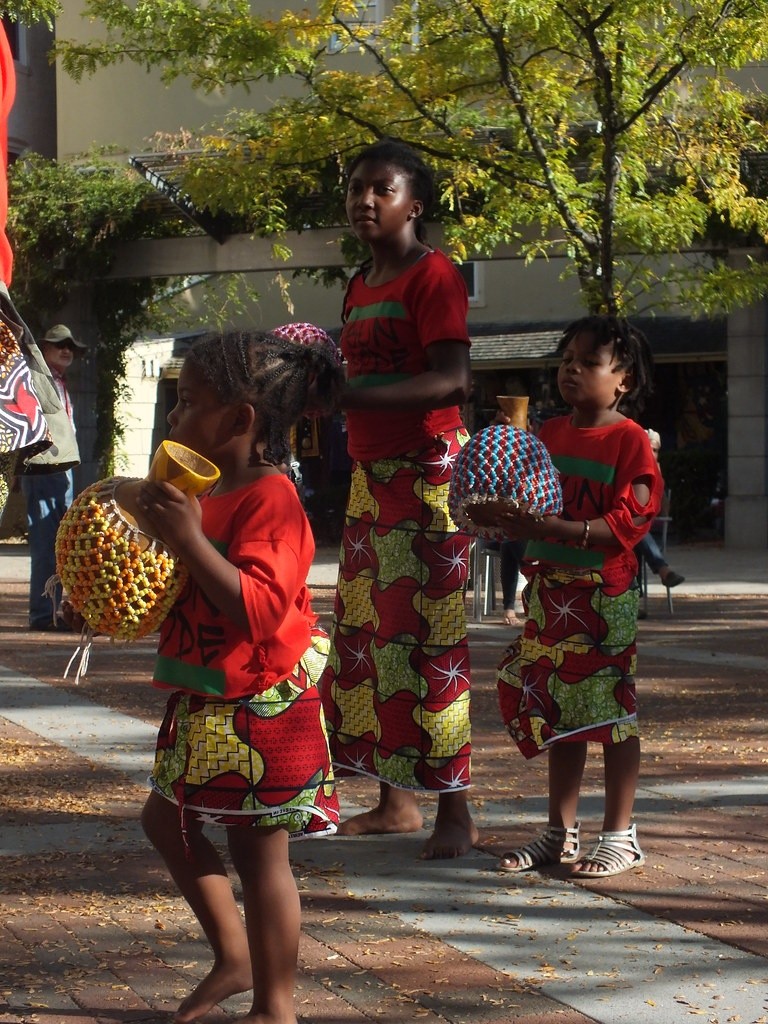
[644,428,661,447]
[38,324,89,360]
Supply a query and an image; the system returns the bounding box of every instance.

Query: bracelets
[573,520,590,550]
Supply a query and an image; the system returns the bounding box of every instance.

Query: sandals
[496,820,582,872]
[566,823,646,878]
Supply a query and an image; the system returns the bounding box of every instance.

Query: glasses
[49,341,77,352]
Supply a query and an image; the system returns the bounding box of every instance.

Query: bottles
[464,394,549,526]
[54,438,223,642]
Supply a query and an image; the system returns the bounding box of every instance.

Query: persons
[20,324,89,630]
[494,311,666,879]
[477,532,529,630]
[61,323,343,1024]
[630,428,685,620]
[301,139,483,863]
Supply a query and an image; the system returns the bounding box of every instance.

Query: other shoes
[662,572,685,587]
[503,616,523,626]
[637,609,647,619]
[30,617,73,633]
